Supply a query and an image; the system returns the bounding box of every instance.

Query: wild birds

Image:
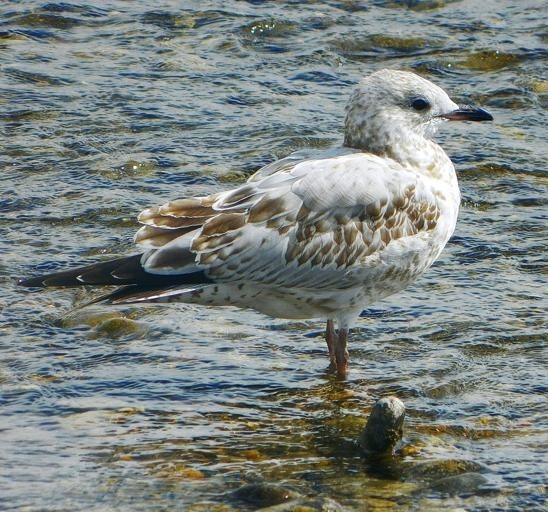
[15,68,495,379]
